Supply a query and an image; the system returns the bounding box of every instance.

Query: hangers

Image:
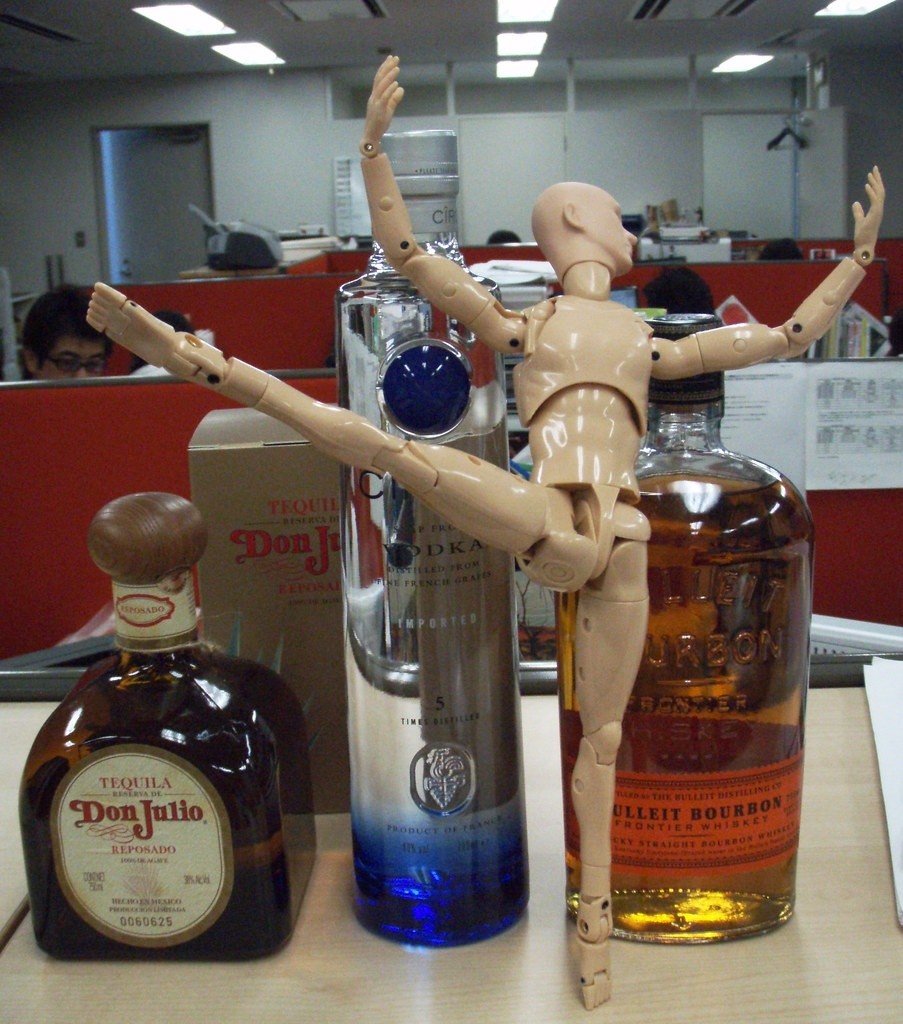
[766,114,809,154]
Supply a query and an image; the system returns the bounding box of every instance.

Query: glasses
[46,351,108,374]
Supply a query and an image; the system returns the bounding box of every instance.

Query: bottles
[335,128,530,948]
[17,493,316,966]
[551,313,814,945]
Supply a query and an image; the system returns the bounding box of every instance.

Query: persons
[85,52,885,1011]
[644,267,717,314]
[18,280,113,378]
